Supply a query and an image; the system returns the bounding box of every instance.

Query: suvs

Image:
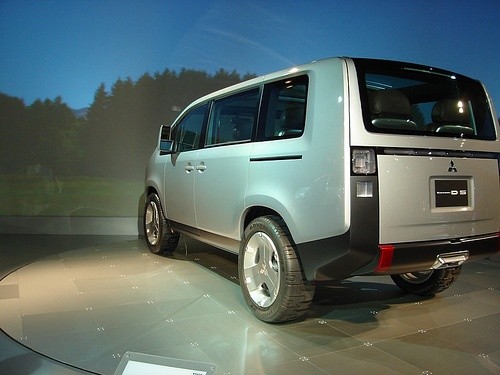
[144,56,499,323]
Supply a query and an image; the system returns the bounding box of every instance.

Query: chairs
[228,124,251,140]
[274,105,304,136]
[426,97,476,134]
[373,89,417,130]
